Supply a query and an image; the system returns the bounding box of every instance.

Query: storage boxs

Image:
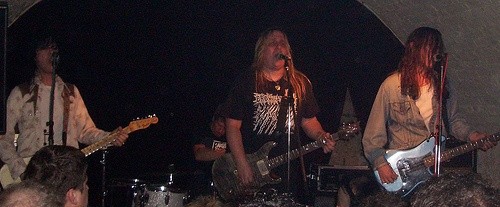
[310,163,369,193]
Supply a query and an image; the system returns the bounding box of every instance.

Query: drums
[132,181,189,207]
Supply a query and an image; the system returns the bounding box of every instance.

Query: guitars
[375,132,500,198]
[0,114,158,190]
[212,120,362,203]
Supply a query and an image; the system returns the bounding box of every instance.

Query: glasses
[422,43,446,52]
[32,44,58,54]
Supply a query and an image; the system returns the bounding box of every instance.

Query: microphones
[434,53,441,62]
[276,53,291,60]
[51,52,60,63]
